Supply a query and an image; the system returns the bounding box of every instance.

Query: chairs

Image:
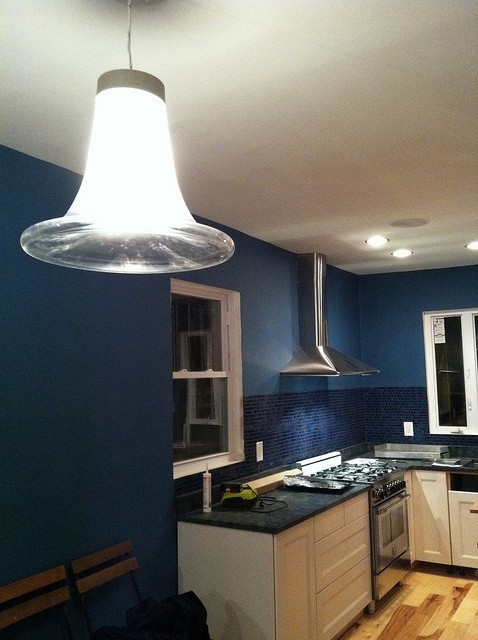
[0,564,71,640]
[70,539,142,640]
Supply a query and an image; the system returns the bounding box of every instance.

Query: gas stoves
[294,449,400,485]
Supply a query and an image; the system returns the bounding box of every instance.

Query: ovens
[371,473,410,601]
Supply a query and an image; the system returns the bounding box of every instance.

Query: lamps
[19,0,237,274]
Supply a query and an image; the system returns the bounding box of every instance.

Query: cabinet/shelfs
[422,310,477,435]
[447,472,478,569]
[273,517,317,640]
[411,468,452,566]
[316,490,374,640]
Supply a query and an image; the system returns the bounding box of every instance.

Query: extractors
[278,281,380,377]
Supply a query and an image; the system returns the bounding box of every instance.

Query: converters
[221,481,242,493]
